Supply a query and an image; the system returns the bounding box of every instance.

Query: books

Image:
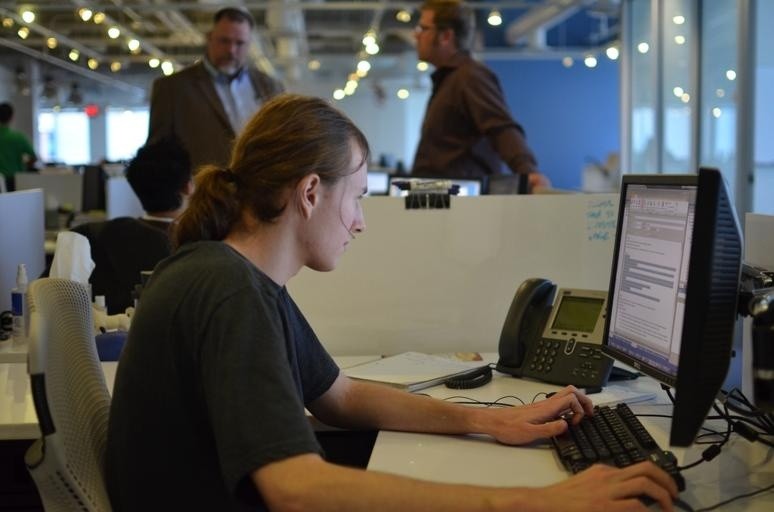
[338,349,487,393]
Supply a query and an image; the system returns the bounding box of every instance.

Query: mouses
[616,496,692,512]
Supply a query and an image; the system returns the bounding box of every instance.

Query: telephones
[495,277,615,388]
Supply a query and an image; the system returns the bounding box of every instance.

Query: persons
[148,8,284,169]
[96,94,680,512]
[124,135,197,274]
[409,2,551,193]
[0,99,38,190]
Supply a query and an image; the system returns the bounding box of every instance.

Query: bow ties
[228,66,244,83]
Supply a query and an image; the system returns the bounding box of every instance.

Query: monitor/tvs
[669,167,774,446]
[602,174,698,387]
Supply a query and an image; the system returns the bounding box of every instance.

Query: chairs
[41,217,178,314]
[22,277,117,512]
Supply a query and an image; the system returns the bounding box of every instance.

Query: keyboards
[550,404,685,491]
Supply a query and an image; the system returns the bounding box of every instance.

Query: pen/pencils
[392,178,460,194]
[546,386,602,399]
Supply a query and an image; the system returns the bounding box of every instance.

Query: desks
[0,354,774,512]
